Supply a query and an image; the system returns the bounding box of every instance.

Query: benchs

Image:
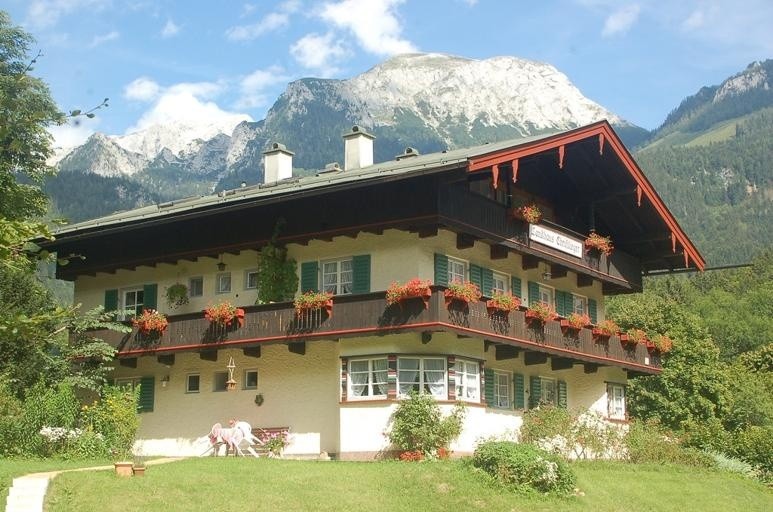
[223,426,289,457]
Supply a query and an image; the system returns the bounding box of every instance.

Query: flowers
[202,299,245,328]
[447,275,482,305]
[384,277,431,309]
[526,300,672,354]
[517,203,542,225]
[585,232,614,257]
[489,288,521,313]
[292,287,332,321]
[130,308,168,336]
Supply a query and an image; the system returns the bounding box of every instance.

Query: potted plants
[113,439,147,480]
[380,386,467,461]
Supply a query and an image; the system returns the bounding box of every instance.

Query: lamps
[158,374,168,388]
[225,356,237,391]
